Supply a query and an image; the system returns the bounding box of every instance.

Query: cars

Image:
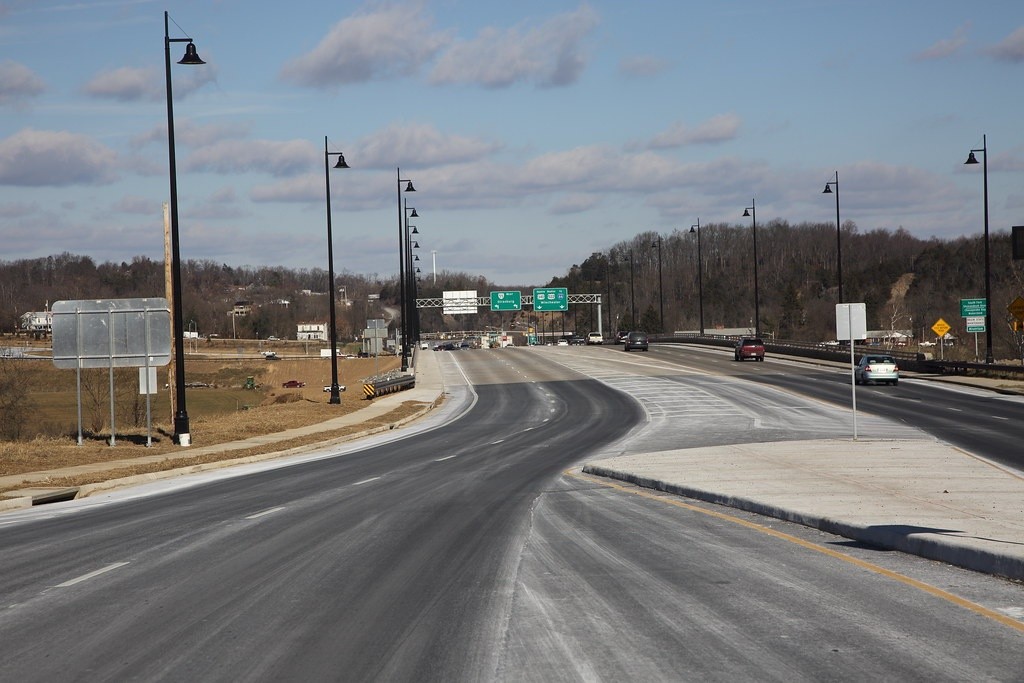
[854,354,899,386]
[358,352,375,358]
[266,355,281,360]
[734,337,766,362]
[432,341,460,352]
[557,339,568,347]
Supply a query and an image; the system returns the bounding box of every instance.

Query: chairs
[884,360,891,363]
[870,360,876,363]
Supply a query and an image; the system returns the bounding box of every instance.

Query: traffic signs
[490,290,521,311]
[533,287,569,311]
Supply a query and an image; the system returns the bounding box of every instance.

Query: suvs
[625,331,648,351]
[614,330,630,345]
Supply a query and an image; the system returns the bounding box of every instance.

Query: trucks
[320,348,347,359]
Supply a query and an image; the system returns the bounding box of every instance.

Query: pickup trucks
[918,340,936,348]
[260,350,276,356]
[323,384,346,393]
[585,332,604,345]
[282,380,306,388]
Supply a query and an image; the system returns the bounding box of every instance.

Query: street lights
[396,166,416,372]
[821,171,847,352]
[403,197,421,358]
[602,261,612,339]
[650,234,664,335]
[624,249,635,331]
[324,137,350,406]
[742,198,761,338]
[964,133,994,365]
[688,217,704,337]
[163,11,208,446]
